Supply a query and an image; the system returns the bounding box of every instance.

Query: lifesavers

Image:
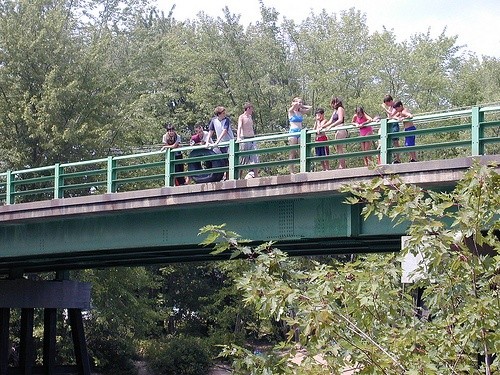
[187,148,224,182]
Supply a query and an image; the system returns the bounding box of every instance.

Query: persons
[376,94,402,165]
[313,109,332,171]
[244,168,255,180]
[204,107,236,181]
[235,102,261,180]
[287,95,314,176]
[316,97,349,170]
[160,123,187,186]
[350,106,374,167]
[393,99,420,163]
[371,115,382,164]
[190,124,224,170]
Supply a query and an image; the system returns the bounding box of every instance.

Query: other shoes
[394,161,401,164]
[409,159,417,162]
